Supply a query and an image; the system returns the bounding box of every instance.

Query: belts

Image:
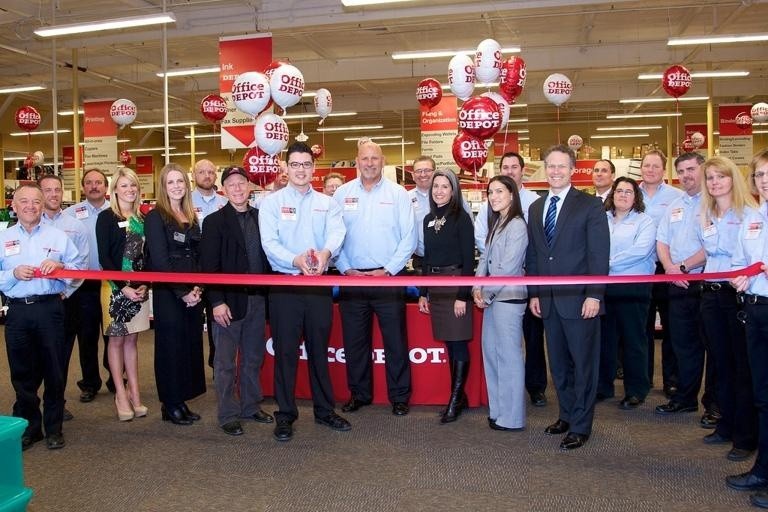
[703,282,733,293]
[745,295,767,306]
[430,265,461,275]
[9,294,60,306]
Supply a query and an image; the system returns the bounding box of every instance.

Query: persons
[188,157,225,390]
[415,167,477,424]
[526,148,611,448]
[403,156,473,271]
[589,144,768,508]
[257,144,353,441]
[469,179,532,431]
[475,152,549,407]
[202,165,274,437]
[322,171,346,198]
[269,164,291,192]
[332,140,419,418]
[1,162,203,451]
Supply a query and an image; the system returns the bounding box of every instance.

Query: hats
[221,167,249,187]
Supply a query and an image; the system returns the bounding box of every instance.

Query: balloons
[272,64,304,109]
[416,78,442,112]
[31,150,45,168]
[201,94,227,124]
[750,102,768,124]
[567,134,583,158]
[544,73,574,109]
[311,144,321,158]
[447,36,526,177]
[263,62,287,84]
[119,151,132,166]
[254,114,289,159]
[682,139,695,154]
[110,99,138,127]
[735,112,752,132]
[313,88,332,119]
[692,132,705,149]
[242,147,280,189]
[23,156,35,167]
[231,71,270,119]
[662,62,693,102]
[16,106,41,131]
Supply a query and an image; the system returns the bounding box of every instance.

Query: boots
[439,360,468,416]
[441,360,469,424]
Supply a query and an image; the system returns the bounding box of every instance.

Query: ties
[545,196,560,249]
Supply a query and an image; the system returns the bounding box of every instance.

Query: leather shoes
[313,414,351,431]
[220,422,243,435]
[392,402,409,416]
[700,410,767,509]
[487,393,698,450]
[161,404,193,426]
[251,411,274,424]
[22,391,95,450]
[342,399,371,413]
[178,405,200,421]
[273,421,292,441]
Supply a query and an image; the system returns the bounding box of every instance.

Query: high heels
[126,389,148,418]
[114,393,135,421]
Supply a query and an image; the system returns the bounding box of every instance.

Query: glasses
[414,169,433,175]
[614,189,634,196]
[287,162,314,170]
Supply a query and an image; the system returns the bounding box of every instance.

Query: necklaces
[426,214,448,232]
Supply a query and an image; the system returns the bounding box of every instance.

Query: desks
[235,308,486,408]
